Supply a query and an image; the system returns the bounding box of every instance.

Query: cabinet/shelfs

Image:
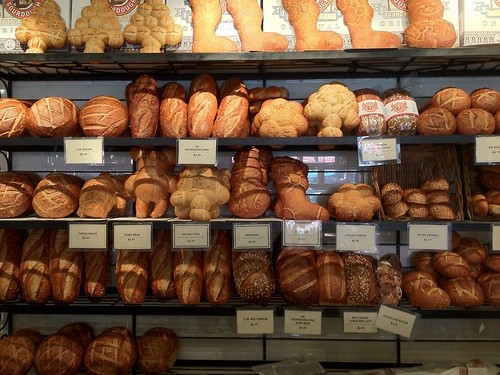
[0,41,500,321]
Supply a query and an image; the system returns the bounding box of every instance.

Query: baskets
[372,144,464,222]
[462,143,500,220]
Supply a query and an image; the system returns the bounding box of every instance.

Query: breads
[382,168,500,219]
[234,230,500,310]
[0,71,251,139]
[248,80,361,138]
[15,0,457,53]
[125,147,379,222]
[0,170,129,219]
[353,88,500,135]
[0,227,233,307]
[1,322,181,375]
